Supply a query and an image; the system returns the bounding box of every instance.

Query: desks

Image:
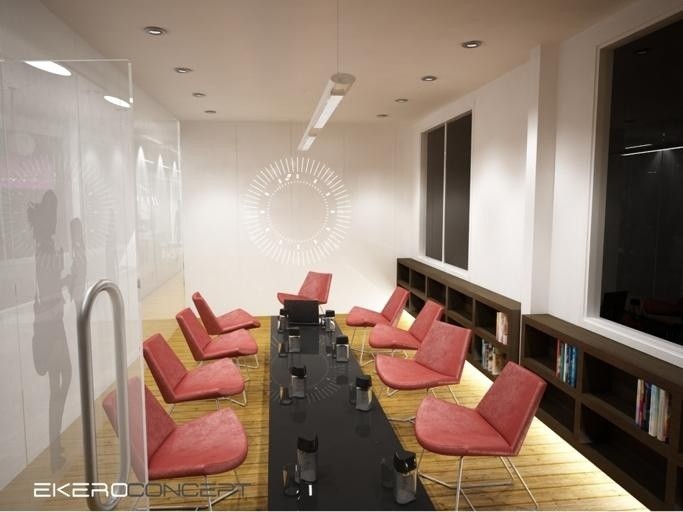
[270,315,437,511]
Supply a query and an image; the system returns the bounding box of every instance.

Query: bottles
[277,308,417,504]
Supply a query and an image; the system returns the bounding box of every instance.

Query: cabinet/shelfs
[396,258,520,383]
[519,314,683,511]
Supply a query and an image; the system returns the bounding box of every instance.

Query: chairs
[102,374,248,511]
[369,299,444,396]
[192,292,261,340]
[142,333,246,418]
[346,287,410,368]
[415,362,547,511]
[376,320,472,424]
[176,307,259,369]
[276,272,332,315]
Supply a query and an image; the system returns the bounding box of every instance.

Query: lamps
[297,72,357,151]
